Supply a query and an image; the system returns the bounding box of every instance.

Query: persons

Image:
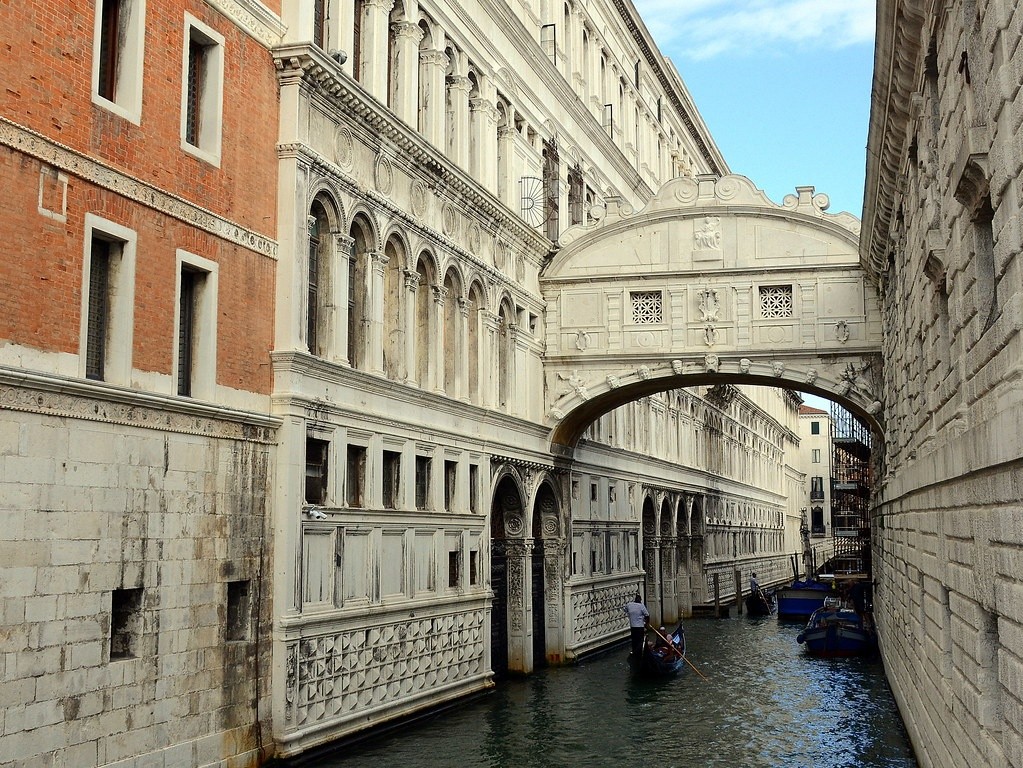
[623,594,651,653]
[846,579,865,629]
[750,573,759,599]
[644,622,676,663]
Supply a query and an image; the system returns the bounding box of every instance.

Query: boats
[775,547,830,622]
[627,610,687,678]
[797,596,870,656]
[745,584,773,615]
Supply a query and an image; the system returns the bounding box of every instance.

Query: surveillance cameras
[319,512,327,520]
[312,511,320,519]
